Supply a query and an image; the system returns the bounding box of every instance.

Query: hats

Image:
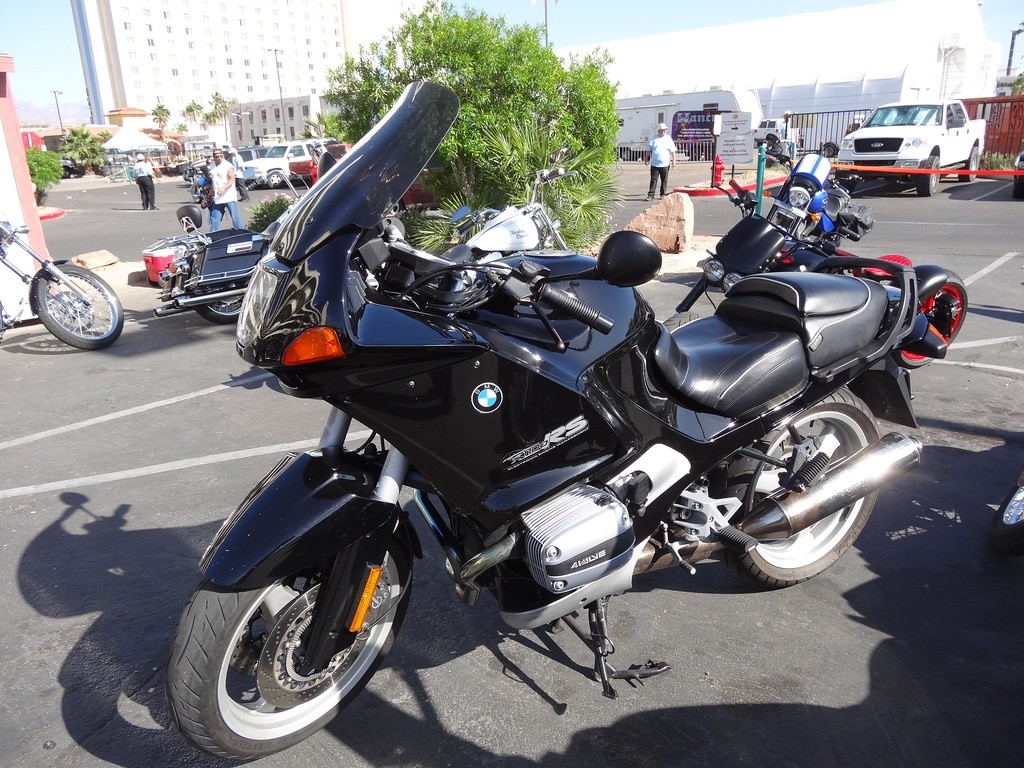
[657,123,668,130]
[136,154,146,160]
[228,147,239,154]
[213,148,223,157]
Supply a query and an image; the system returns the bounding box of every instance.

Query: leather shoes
[660,194,664,199]
[646,195,654,200]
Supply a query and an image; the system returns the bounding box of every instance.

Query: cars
[185,144,271,183]
[1012,137,1024,200]
[60,160,76,178]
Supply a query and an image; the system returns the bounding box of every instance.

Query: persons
[133,153,163,211]
[204,148,242,232]
[228,147,250,202]
[644,123,677,200]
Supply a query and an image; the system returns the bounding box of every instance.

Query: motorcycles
[184,168,213,205]
[152,152,406,323]
[757,133,876,256]
[0,220,126,351]
[165,74,920,763]
[382,147,572,313]
[662,179,968,369]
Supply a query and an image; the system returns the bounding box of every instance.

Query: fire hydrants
[710,156,727,185]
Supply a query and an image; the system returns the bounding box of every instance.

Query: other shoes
[238,198,250,202]
[194,192,200,196]
[143,207,158,210]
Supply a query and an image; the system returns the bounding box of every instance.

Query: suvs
[243,138,337,190]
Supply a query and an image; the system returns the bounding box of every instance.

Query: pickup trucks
[289,142,356,182]
[834,98,986,197]
[106,148,129,165]
[752,119,788,146]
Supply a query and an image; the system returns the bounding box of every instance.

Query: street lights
[48,91,64,133]
[267,49,288,140]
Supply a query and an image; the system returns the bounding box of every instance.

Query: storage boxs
[141,244,190,281]
